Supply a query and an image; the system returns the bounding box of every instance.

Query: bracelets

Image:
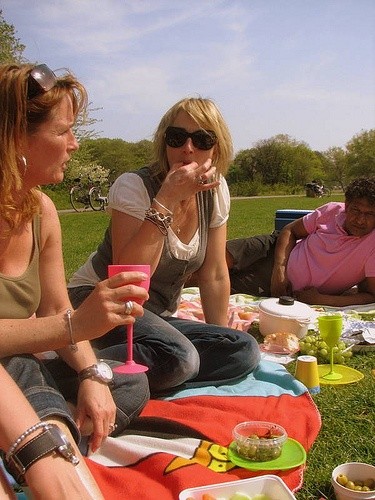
[5,422,49,461]
[145,198,174,236]
[67,309,76,350]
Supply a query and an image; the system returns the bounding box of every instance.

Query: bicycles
[69,168,99,213]
[88,169,115,211]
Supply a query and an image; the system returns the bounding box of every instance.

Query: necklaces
[173,198,193,233]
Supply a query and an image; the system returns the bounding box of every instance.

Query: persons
[184,177,375,306]
[67,98,261,392]
[0,363,92,500]
[0,63,149,500]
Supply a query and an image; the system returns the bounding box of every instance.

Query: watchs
[8,424,79,486]
[78,359,113,384]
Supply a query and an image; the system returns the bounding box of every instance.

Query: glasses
[27,63,57,98]
[165,127,216,150]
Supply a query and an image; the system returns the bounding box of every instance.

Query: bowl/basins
[331,462,375,500]
[231,420,289,462]
[178,474,297,500]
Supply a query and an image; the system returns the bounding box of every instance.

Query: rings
[125,301,134,314]
[198,176,204,184]
[109,425,115,432]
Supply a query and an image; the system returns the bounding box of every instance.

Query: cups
[293,355,321,395]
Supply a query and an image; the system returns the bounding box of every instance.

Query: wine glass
[316,316,343,381]
[107,264,151,375]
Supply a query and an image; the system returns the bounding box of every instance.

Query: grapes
[299,333,353,363]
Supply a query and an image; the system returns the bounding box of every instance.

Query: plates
[259,343,300,355]
[227,437,306,471]
[317,363,364,385]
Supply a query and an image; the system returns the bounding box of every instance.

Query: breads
[263,332,299,351]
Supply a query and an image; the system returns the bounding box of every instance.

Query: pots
[258,295,314,341]
